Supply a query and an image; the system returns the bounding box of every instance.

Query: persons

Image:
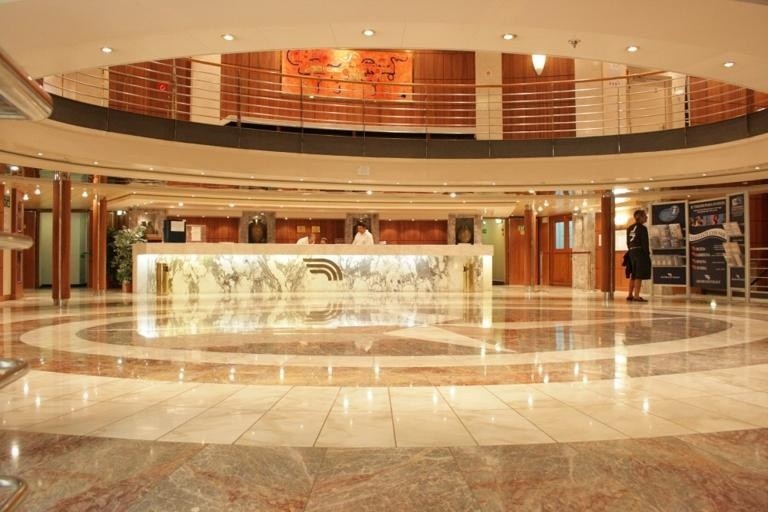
[297,234,316,244]
[321,238,328,244]
[627,210,651,302]
[352,224,374,245]
[712,214,720,224]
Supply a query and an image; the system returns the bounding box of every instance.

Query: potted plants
[110,226,148,292]
